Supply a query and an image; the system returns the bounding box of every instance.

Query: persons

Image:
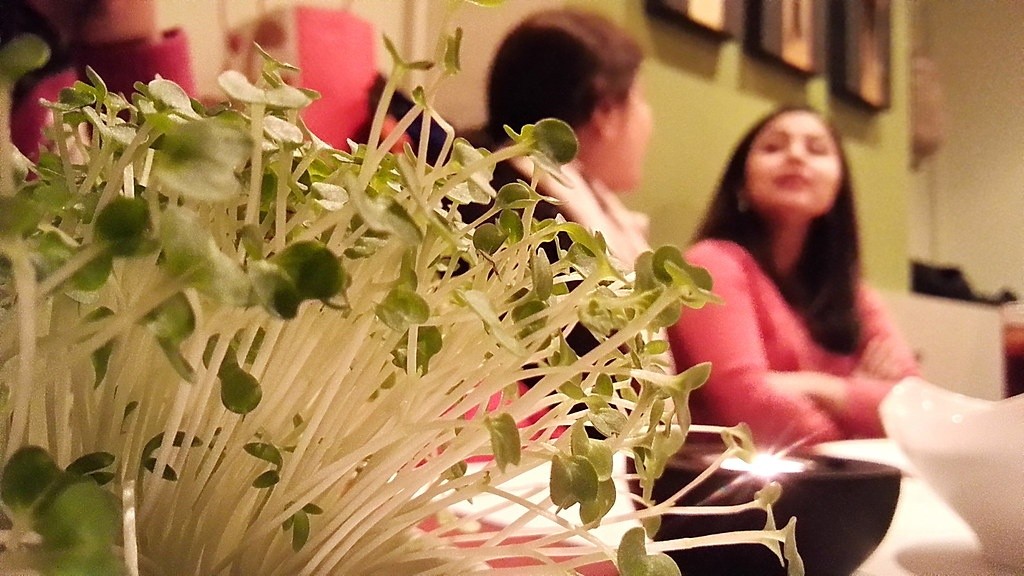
[1,1,201,194]
[446,6,681,441]
[662,107,925,456]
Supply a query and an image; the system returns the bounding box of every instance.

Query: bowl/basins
[625,440,904,576]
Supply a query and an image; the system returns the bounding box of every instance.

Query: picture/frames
[836,0,893,109]
[751,1,824,79]
[649,0,733,40]
